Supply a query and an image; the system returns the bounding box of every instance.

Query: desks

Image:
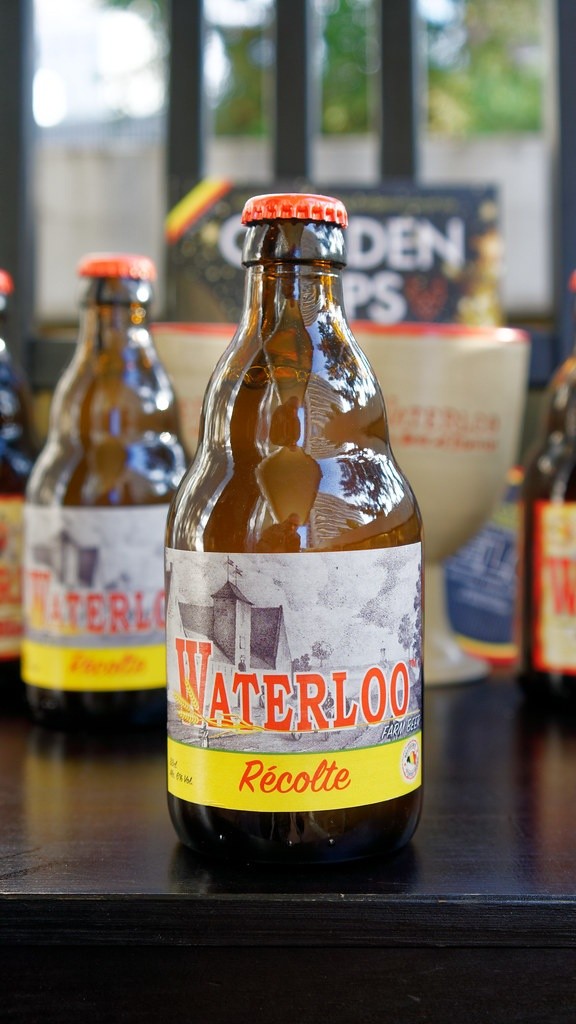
[0,661,574,1024]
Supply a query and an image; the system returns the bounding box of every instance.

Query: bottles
[159,194,427,863]
[516,273,576,745]
[0,269,41,715]
[20,252,187,754]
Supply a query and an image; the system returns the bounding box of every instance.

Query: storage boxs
[532,501,576,678]
